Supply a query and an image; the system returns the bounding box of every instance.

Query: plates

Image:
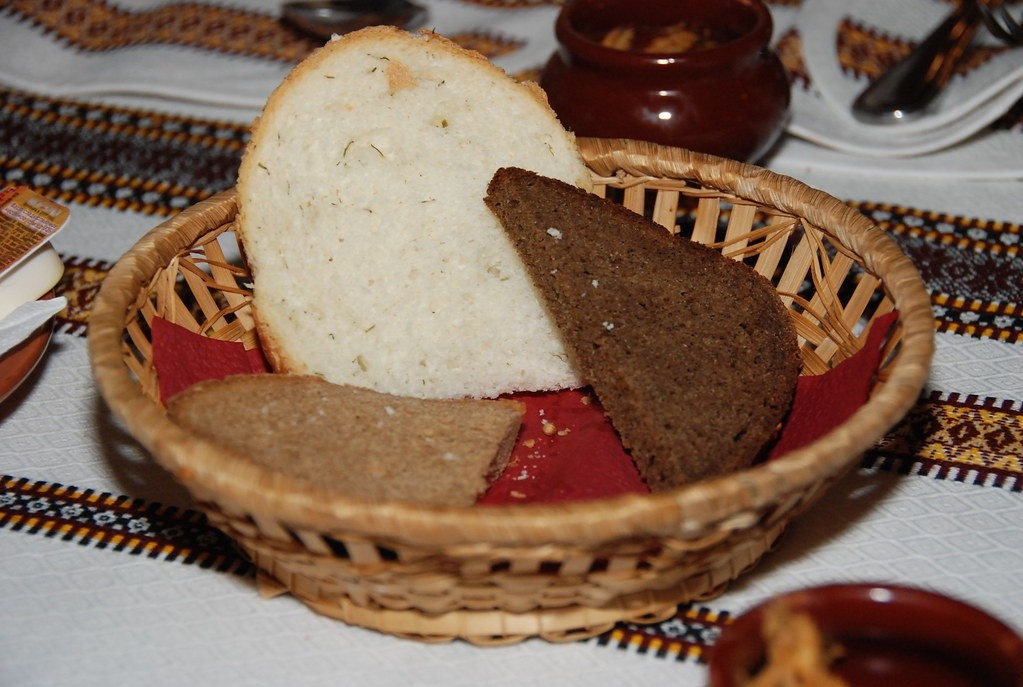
[0,289,56,402]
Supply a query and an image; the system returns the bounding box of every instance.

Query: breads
[160,373,527,508]
[485,168,801,494]
[233,25,602,401]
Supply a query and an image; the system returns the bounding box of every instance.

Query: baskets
[90,137,933,646]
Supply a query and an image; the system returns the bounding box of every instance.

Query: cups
[708,581,1023,687]
[537,0,791,165]
